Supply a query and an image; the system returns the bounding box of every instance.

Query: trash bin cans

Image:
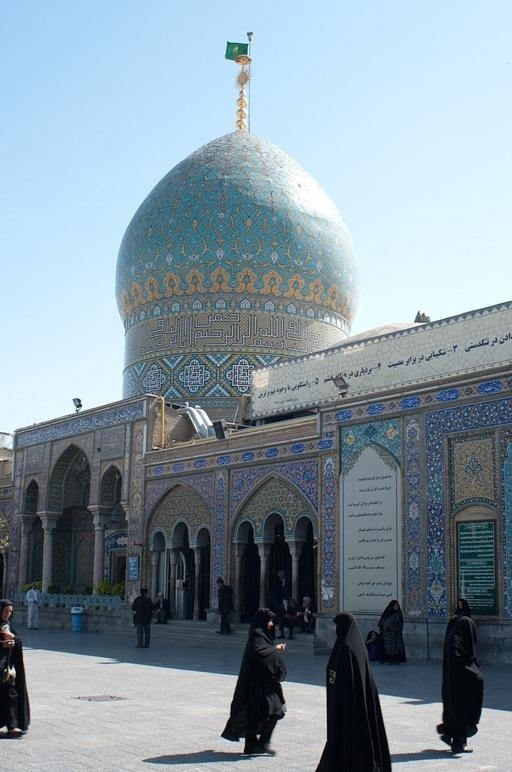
[71,607,84,632]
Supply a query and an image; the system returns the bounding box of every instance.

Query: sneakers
[277,634,295,640]
[1,730,26,738]
[440,733,474,753]
[244,740,276,755]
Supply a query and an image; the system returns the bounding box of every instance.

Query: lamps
[333,375,347,397]
[73,398,82,414]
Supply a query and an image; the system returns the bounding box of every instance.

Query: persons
[442,614,484,755]
[295,595,317,634]
[214,576,234,635]
[314,611,392,772]
[275,565,292,603]
[25,582,41,630]
[443,597,472,636]
[363,630,383,662]
[153,591,174,625]
[131,587,154,648]
[375,599,407,668]
[221,605,288,758]
[0,598,31,739]
[434,614,461,745]
[277,596,298,639]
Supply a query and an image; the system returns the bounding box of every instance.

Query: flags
[224,41,248,62]
